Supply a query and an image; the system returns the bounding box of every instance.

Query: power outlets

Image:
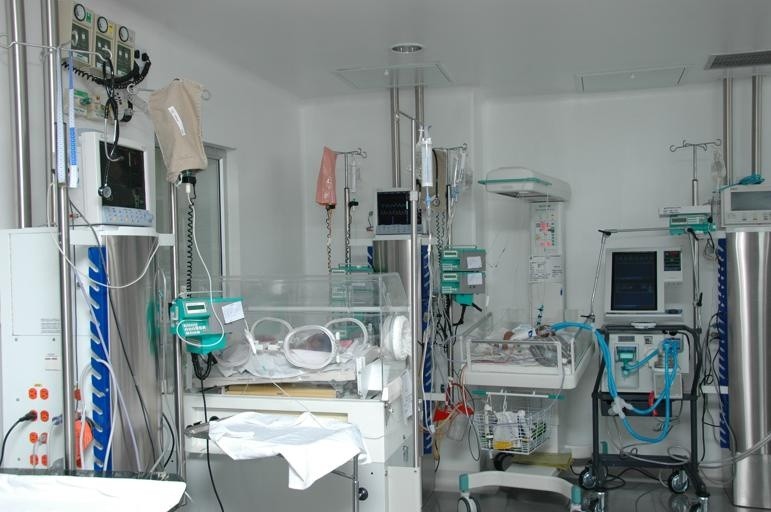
[26,385,52,467]
